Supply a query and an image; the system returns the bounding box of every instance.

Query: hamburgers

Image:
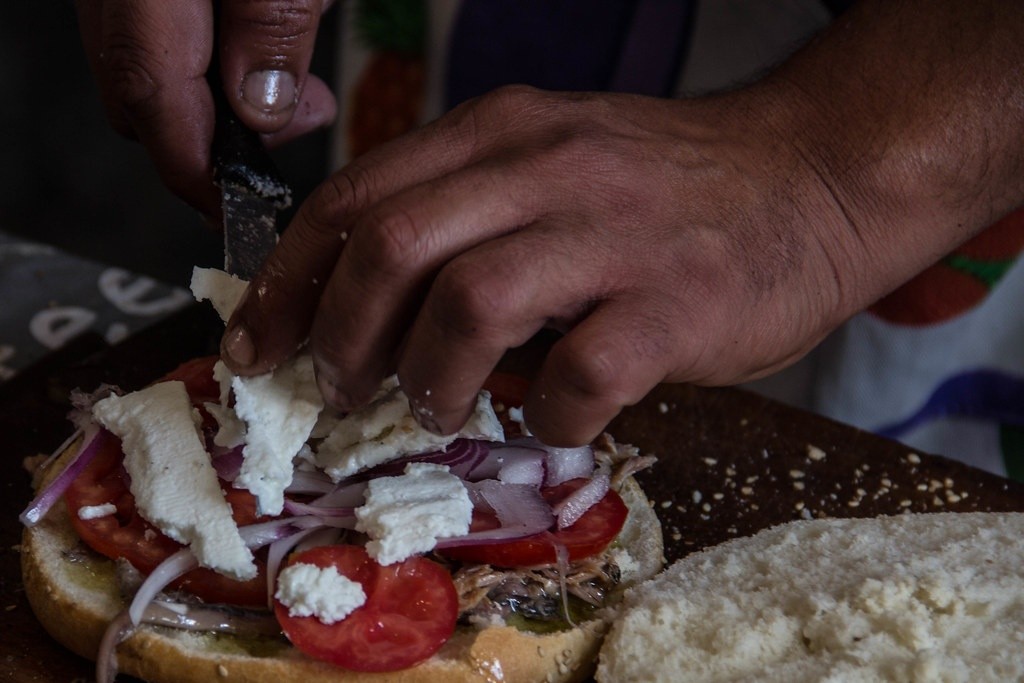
[20,269,1022,680]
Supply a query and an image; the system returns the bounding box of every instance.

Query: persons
[71,1,1024,483]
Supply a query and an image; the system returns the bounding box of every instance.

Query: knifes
[222,93,306,285]
